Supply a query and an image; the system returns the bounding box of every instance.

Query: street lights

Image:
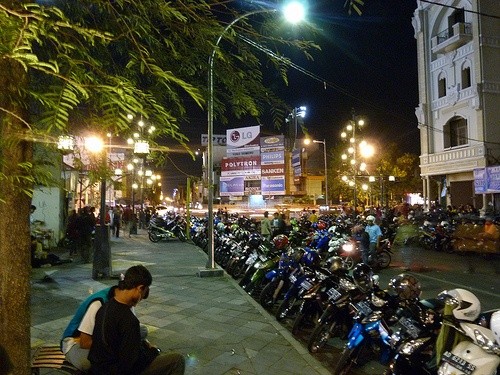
[207,1,306,270]
[338,105,376,216]
[126,121,155,230]
[303,138,328,206]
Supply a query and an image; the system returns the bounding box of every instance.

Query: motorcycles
[145,208,500,375]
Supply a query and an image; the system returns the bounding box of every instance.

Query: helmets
[353,262,373,282]
[248,233,262,249]
[325,256,343,272]
[300,247,318,267]
[217,222,225,231]
[437,288,481,321]
[388,273,422,302]
[273,234,289,249]
[230,224,251,240]
[366,215,375,221]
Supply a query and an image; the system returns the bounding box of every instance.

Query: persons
[139,210,151,229]
[408,210,414,220]
[31,235,49,267]
[437,203,477,214]
[68,205,96,260]
[88,265,186,375]
[397,212,405,223]
[214,207,229,220]
[98,205,123,238]
[261,211,286,239]
[364,216,384,265]
[353,225,371,269]
[302,209,319,229]
[60,284,152,374]
